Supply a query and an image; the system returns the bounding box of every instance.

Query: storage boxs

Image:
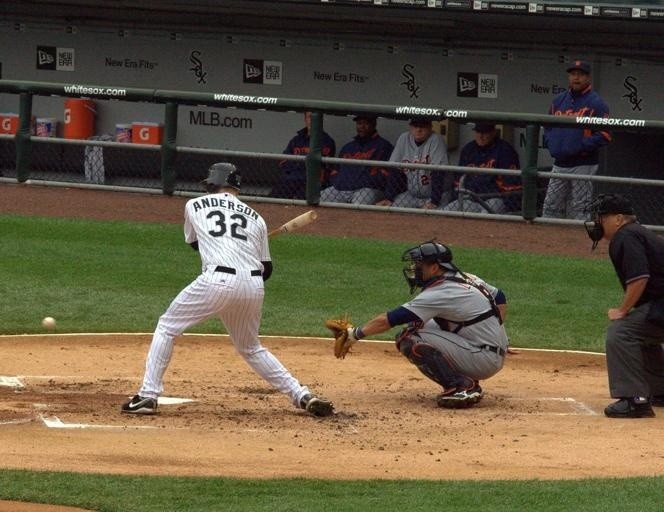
[0,113,35,137]
[131,122,164,144]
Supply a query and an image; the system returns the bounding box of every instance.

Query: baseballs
[43,317,55,330]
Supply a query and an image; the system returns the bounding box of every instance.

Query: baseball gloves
[326,319,355,359]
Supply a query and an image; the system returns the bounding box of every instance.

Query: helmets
[200,162,243,192]
[401,241,459,294]
[580,192,637,251]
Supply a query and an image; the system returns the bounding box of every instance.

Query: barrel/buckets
[62,97,99,139]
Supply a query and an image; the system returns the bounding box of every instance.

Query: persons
[269,112,336,199]
[586,194,661,417]
[321,114,394,205]
[124,161,333,415]
[542,60,611,219]
[444,120,518,213]
[377,119,454,211]
[346,239,508,405]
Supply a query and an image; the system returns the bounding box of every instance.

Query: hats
[471,120,497,134]
[408,117,433,126]
[566,59,593,75]
[352,113,378,122]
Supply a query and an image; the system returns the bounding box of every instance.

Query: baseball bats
[268,210,317,238]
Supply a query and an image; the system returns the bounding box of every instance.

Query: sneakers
[120,393,158,414]
[603,396,663,419]
[435,383,487,409]
[298,392,334,417]
